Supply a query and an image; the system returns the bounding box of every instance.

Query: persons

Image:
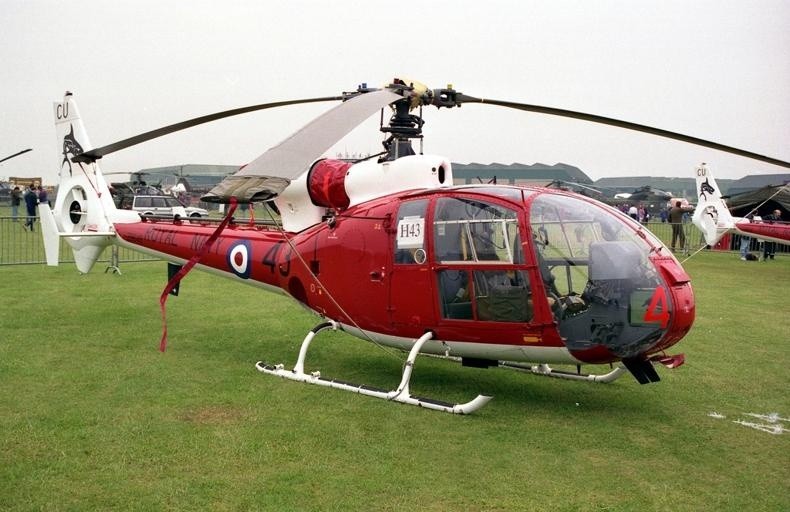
[740,208,759,261]
[22,187,39,233]
[38,185,47,202]
[23,182,34,232]
[10,186,22,222]
[615,202,650,227]
[667,201,695,253]
[763,209,783,261]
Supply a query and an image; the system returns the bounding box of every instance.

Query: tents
[704,184,790,251]
